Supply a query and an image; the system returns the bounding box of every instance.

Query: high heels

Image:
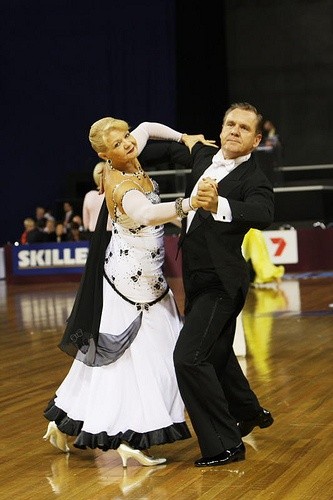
[117,443,167,468]
[121,465,166,496]
[42,421,70,453]
[45,453,70,494]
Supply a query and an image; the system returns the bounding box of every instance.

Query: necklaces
[110,160,148,182]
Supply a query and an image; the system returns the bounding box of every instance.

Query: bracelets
[174,197,189,220]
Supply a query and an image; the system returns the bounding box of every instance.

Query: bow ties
[212,154,235,171]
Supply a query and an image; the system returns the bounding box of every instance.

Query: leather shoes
[194,442,246,466]
[237,407,273,438]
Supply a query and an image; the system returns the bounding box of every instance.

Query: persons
[90,99,275,471]
[43,116,220,467]
[80,161,113,239]
[15,201,87,242]
[259,119,283,159]
